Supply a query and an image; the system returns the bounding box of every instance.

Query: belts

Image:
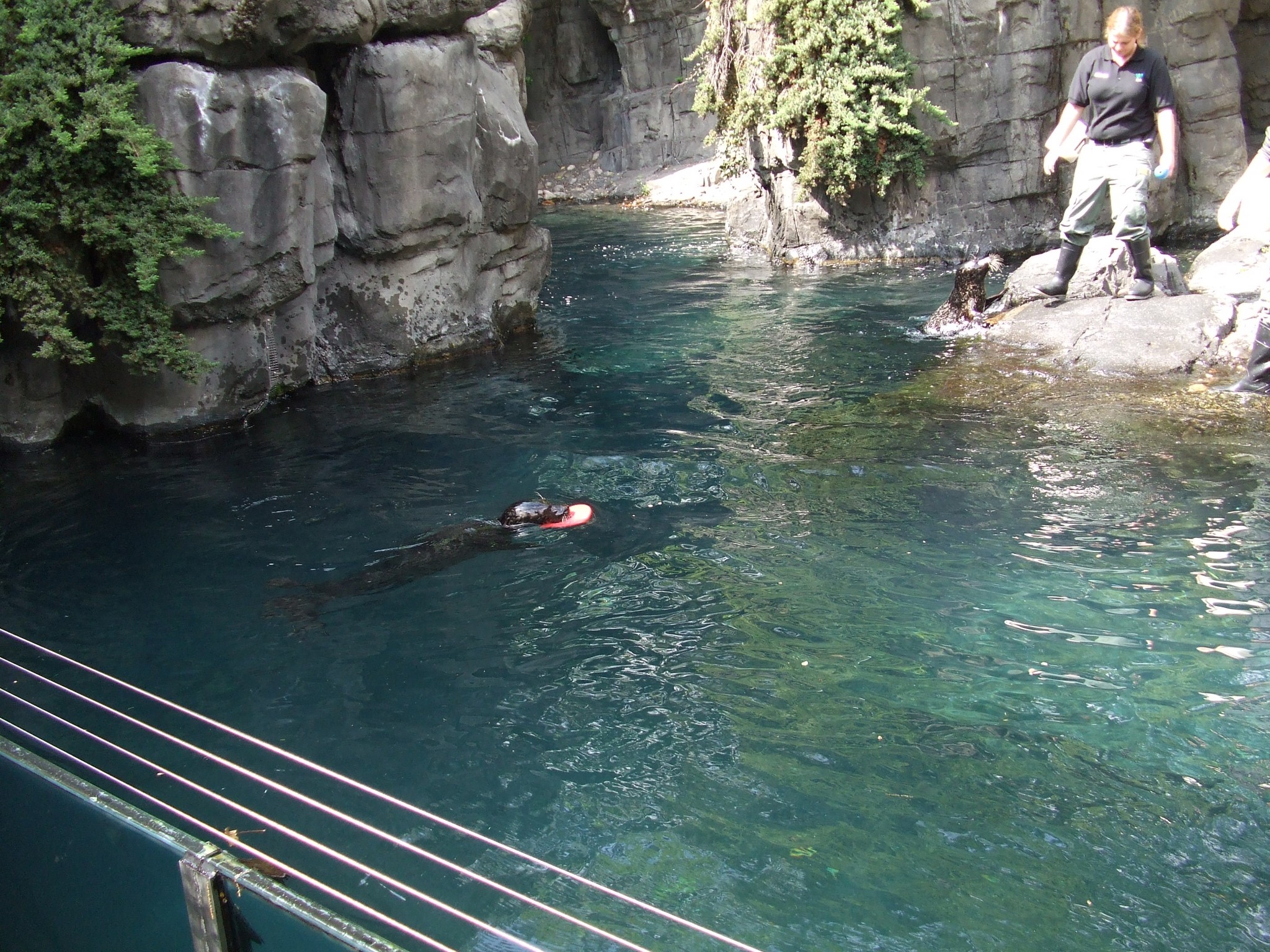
[1094,134,1143,147]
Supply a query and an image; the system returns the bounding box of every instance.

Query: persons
[1031,6,1176,300]
[1216,126,1270,396]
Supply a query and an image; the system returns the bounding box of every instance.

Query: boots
[1212,318,1270,396]
[1031,238,1084,300]
[1124,236,1152,299]
